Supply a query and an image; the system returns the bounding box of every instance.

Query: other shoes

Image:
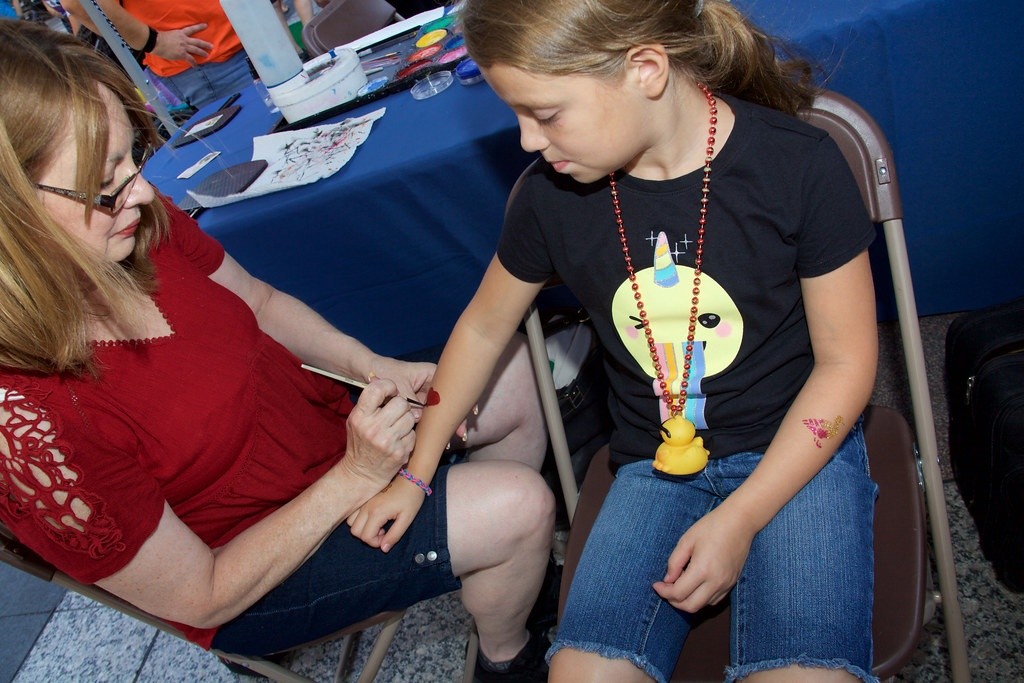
[472,565,563,632]
[464,629,552,683]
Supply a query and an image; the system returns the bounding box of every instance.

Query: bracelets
[398,468,432,496]
[142,26,158,53]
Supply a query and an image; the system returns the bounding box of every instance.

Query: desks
[139,0,1024,377]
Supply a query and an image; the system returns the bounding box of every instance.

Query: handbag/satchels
[944,297,1024,594]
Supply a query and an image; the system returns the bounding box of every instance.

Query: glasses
[30,155,148,214]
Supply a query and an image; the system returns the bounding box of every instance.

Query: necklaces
[610,81,718,475]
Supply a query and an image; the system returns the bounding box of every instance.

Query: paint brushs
[360,52,401,75]
[298,362,425,409]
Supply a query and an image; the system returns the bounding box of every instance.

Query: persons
[0,0,454,111]
[346,0,878,683]
[0,17,563,683]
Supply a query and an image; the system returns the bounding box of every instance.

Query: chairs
[503,86,972,683]
[0,520,482,683]
